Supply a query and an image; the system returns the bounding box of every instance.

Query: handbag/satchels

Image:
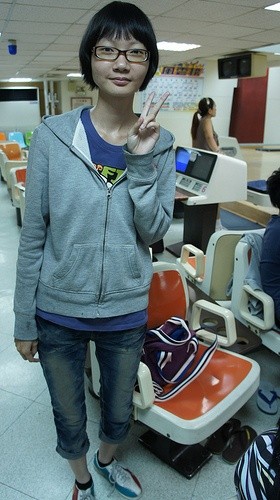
[141,316,198,388]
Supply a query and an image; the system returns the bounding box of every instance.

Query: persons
[257,166,280,331]
[12,0,178,500]
[190,97,223,153]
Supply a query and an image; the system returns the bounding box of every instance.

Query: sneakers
[72,473,94,500]
[93,449,142,500]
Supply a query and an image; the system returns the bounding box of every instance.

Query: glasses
[92,46,150,63]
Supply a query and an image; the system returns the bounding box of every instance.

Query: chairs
[1,132,280,479]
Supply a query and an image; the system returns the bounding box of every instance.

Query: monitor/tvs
[175,146,218,183]
[217,52,268,79]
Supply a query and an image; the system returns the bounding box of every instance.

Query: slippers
[204,416,241,454]
[223,424,258,464]
